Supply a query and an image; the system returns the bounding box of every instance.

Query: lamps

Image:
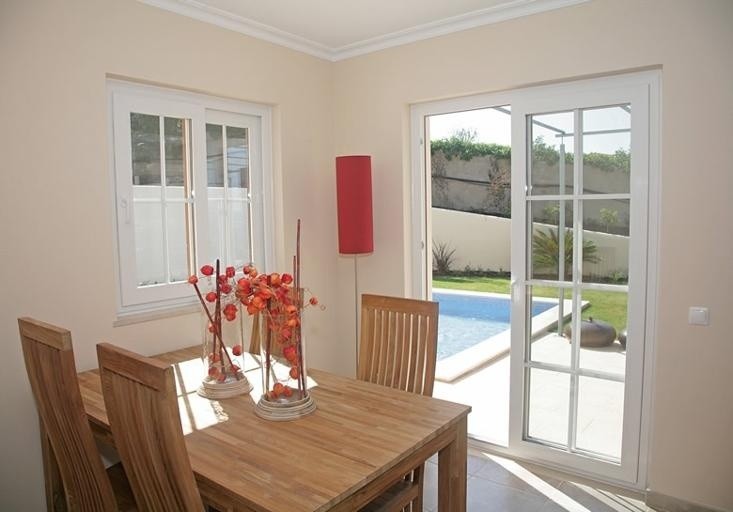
[333,153,374,378]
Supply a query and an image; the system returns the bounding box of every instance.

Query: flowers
[239,263,327,400]
[191,258,244,383]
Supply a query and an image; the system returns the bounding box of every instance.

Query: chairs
[96,339,207,512]
[17,315,119,512]
[249,287,304,359]
[359,290,436,512]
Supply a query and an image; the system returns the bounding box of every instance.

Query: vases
[256,316,315,422]
[215,306,253,400]
